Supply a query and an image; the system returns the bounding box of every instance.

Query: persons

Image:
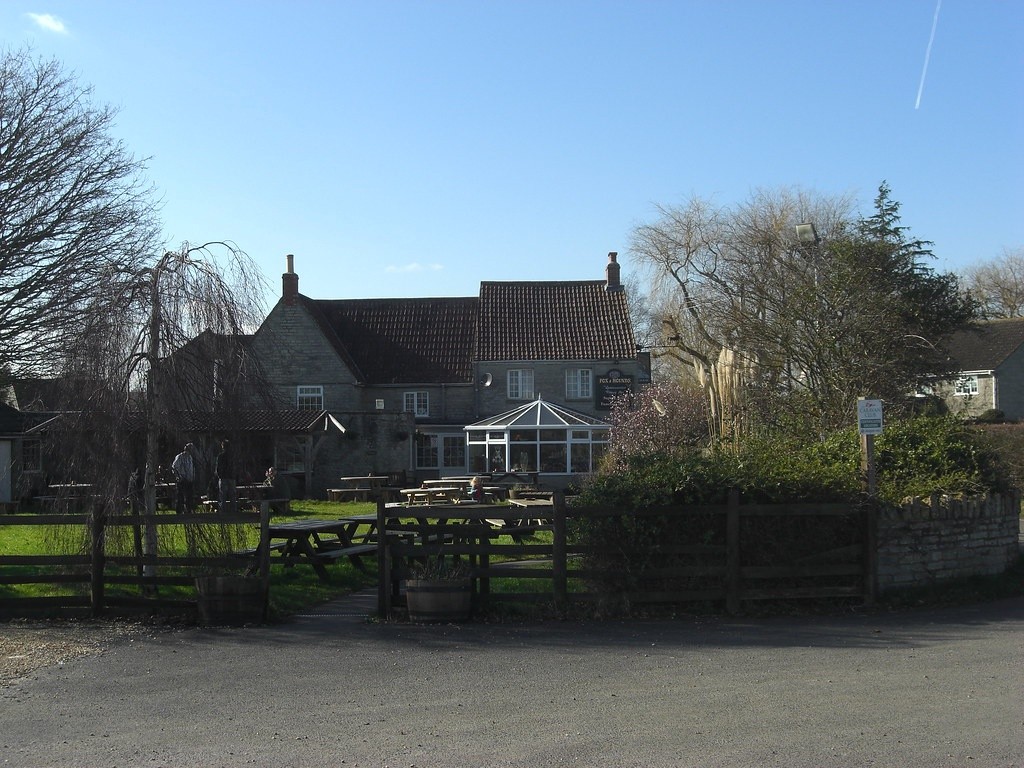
[128,467,145,511]
[262,467,292,513]
[215,440,244,513]
[172,443,196,515]
[471,476,491,504]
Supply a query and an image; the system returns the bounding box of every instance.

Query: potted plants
[405,554,472,622]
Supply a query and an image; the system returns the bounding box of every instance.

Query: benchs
[30,496,291,517]
[327,487,371,505]
[486,519,507,529]
[233,531,461,565]
[399,487,506,506]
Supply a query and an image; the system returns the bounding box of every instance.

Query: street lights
[796,222,826,443]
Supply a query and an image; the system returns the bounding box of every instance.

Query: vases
[196,575,271,626]
[509,490,520,498]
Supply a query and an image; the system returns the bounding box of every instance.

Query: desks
[423,480,470,503]
[236,485,281,514]
[48,485,92,511]
[442,476,491,494]
[341,476,389,502]
[334,515,414,546]
[155,483,177,508]
[242,520,356,580]
[519,491,554,500]
[508,497,553,526]
